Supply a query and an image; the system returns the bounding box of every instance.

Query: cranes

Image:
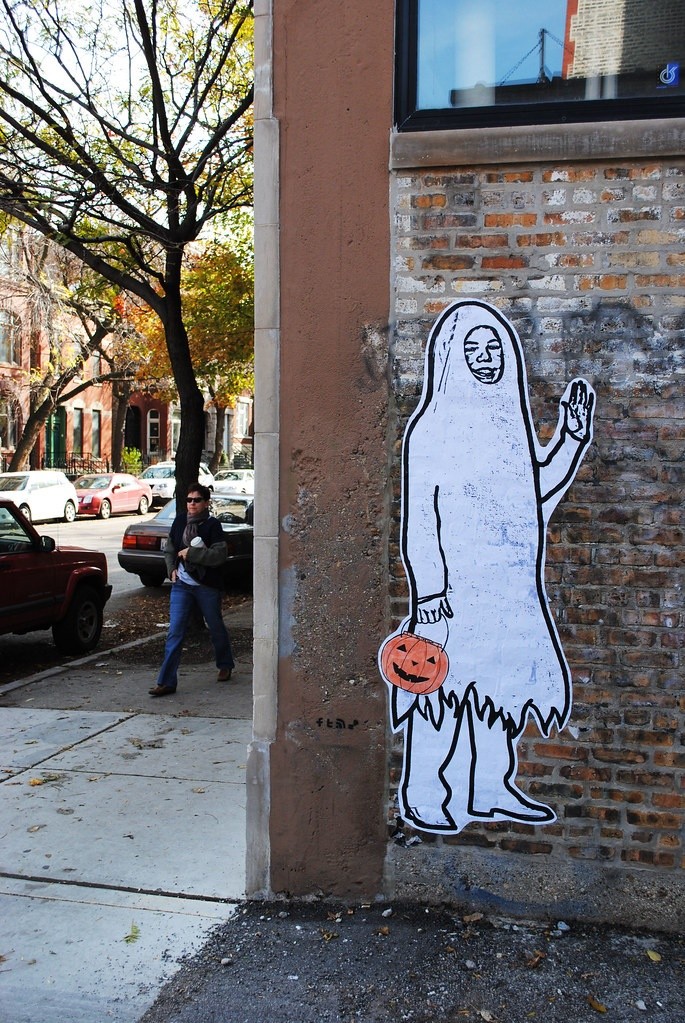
[494,29,603,86]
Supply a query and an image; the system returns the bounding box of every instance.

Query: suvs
[138,461,214,506]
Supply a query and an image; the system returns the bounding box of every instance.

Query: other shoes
[148,684,176,696]
[218,667,232,681]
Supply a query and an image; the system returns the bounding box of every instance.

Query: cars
[0,497,113,654]
[213,469,254,494]
[73,473,152,519]
[117,495,253,587]
[0,471,78,529]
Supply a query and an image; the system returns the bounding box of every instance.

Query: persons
[149,483,235,694]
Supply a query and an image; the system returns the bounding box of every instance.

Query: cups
[190,537,207,551]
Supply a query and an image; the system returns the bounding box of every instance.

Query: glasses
[186,496,203,503]
